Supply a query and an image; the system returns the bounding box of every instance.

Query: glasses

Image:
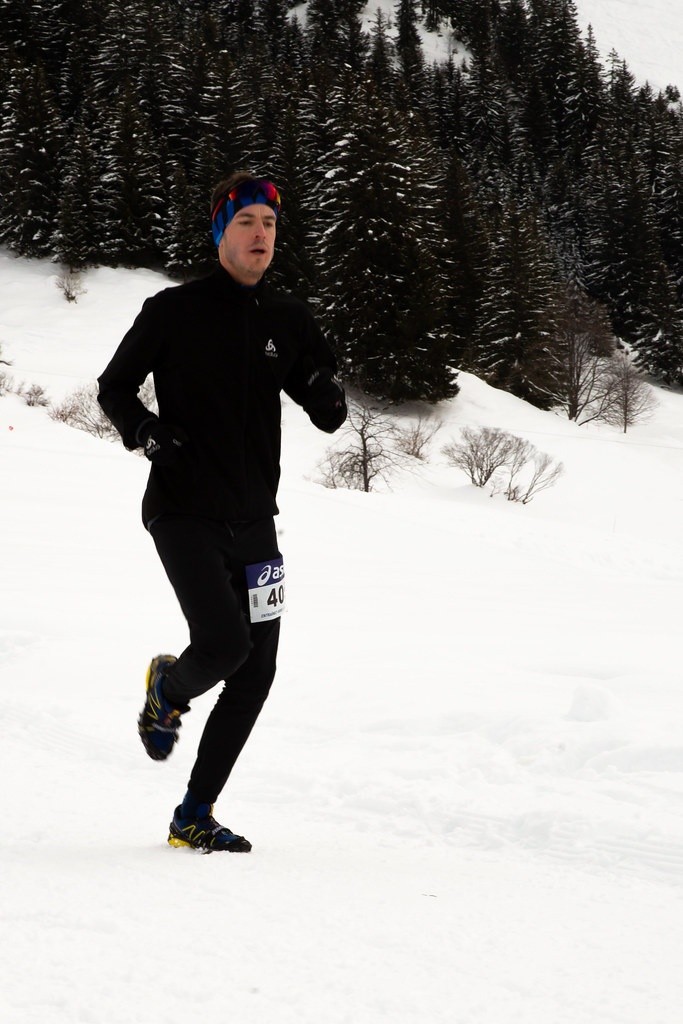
[212,179,281,222]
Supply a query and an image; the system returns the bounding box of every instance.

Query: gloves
[303,367,345,424]
[144,422,187,467]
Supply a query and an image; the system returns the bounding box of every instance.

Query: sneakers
[167,804,252,853]
[138,655,190,761]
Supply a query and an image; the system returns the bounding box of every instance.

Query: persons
[97,171,347,854]
